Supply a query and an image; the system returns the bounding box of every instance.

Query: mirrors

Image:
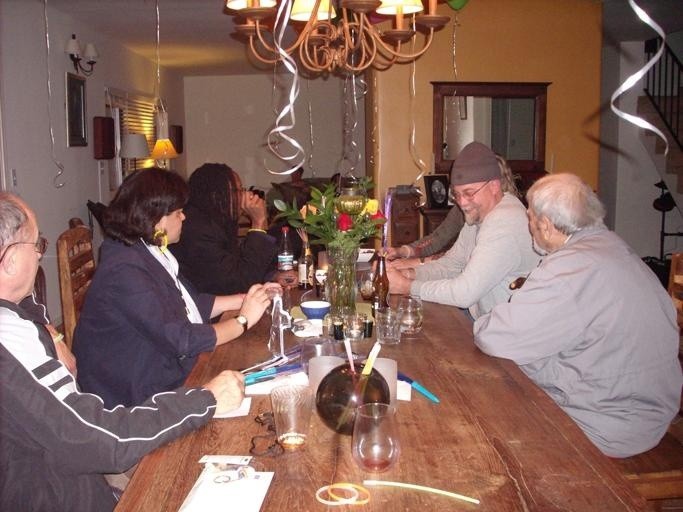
[431,82,552,174]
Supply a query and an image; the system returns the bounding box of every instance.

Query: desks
[113,248,658,512]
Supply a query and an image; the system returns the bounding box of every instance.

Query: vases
[328,247,358,315]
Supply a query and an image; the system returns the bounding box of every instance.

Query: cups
[263,248,424,344]
[308,357,345,400]
[269,384,313,448]
[361,357,398,408]
[352,402,404,473]
[301,337,341,379]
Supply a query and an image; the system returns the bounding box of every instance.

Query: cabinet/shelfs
[389,187,423,248]
[420,190,597,254]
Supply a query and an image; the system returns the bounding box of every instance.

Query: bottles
[298,240,314,290]
[277,227,294,283]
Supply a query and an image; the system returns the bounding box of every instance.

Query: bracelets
[52,333,65,344]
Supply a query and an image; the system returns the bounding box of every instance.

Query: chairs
[70,218,95,294]
[57,225,96,352]
[608,252,683,512]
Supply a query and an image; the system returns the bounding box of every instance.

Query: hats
[449,140,504,187]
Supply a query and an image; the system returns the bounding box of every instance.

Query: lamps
[226,0,451,73]
[152,138,178,171]
[65,34,96,76]
[119,133,150,172]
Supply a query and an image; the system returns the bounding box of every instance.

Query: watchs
[232,313,248,336]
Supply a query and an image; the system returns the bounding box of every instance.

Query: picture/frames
[65,70,88,147]
[458,96,467,120]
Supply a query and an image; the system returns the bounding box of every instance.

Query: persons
[70,166,285,411]
[473,173,683,460]
[288,164,312,194]
[385,141,541,322]
[0,191,246,512]
[375,153,521,261]
[167,162,278,294]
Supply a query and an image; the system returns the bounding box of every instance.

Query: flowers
[267,176,388,255]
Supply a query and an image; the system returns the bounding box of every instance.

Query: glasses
[0,235,49,265]
[447,180,495,203]
